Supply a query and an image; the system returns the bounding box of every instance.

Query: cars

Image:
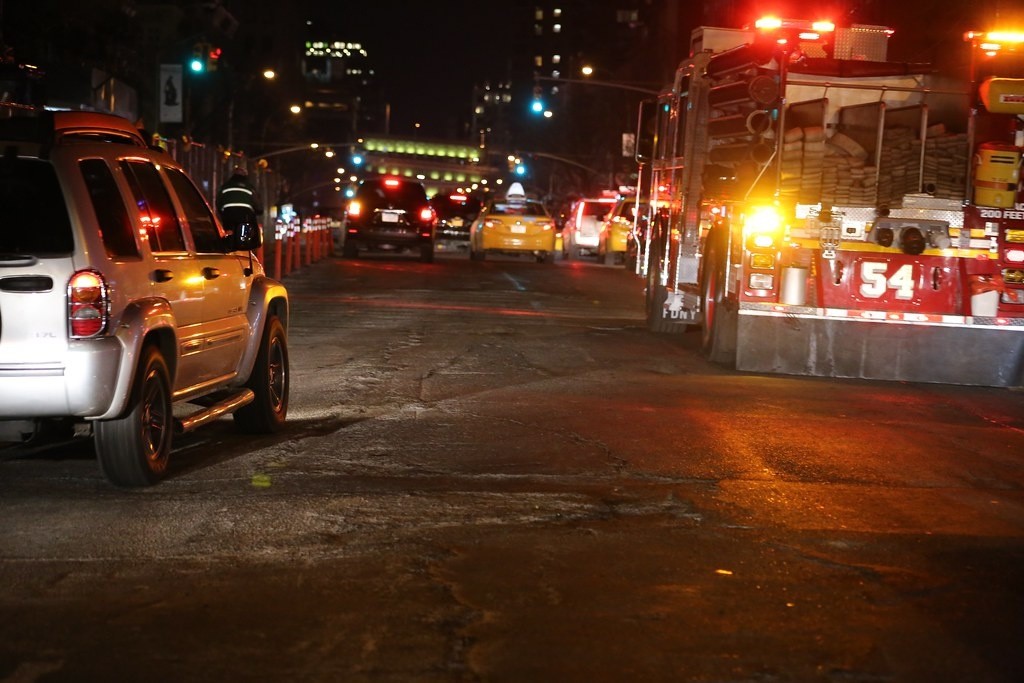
[562,196,618,260]
[467,182,556,264]
[596,195,650,267]
[425,192,483,242]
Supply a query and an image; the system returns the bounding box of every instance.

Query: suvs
[342,174,437,261]
[0,101,291,491]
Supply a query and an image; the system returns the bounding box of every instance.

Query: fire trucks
[622,14,1024,389]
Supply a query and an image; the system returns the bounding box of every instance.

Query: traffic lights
[207,44,219,71]
[514,157,530,177]
[531,86,545,115]
[190,42,204,74]
